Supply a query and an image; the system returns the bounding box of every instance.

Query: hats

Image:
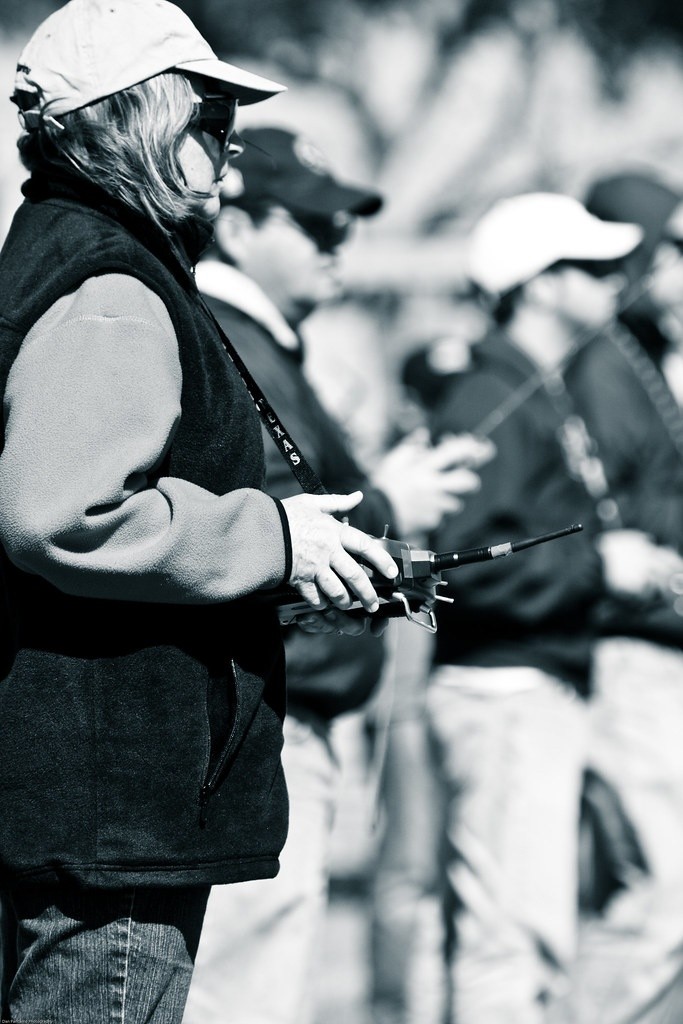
[471,192,644,293]
[228,129,383,217]
[17,0,289,133]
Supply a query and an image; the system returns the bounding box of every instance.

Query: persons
[184,123,682,1024]
[0,0,398,1024]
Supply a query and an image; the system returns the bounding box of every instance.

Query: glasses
[269,209,348,246]
[564,259,624,277]
[188,94,240,153]
